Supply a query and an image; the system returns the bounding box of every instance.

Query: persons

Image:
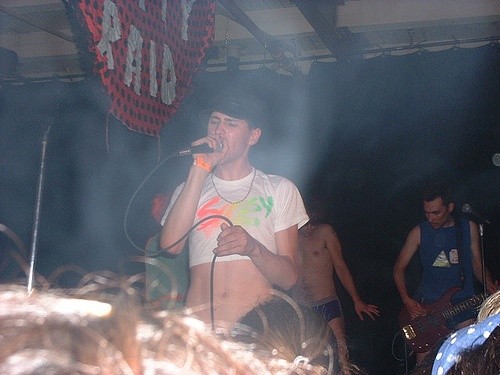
[297,203,381,375]
[160,99,309,336]
[391,188,497,366]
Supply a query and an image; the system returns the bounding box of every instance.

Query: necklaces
[211,169,256,203]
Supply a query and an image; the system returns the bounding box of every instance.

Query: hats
[199,96,261,132]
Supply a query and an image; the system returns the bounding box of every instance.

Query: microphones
[461,203,493,225]
[176,142,223,157]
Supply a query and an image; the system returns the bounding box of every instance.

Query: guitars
[397,283,496,353]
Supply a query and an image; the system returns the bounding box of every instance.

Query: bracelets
[192,159,211,172]
